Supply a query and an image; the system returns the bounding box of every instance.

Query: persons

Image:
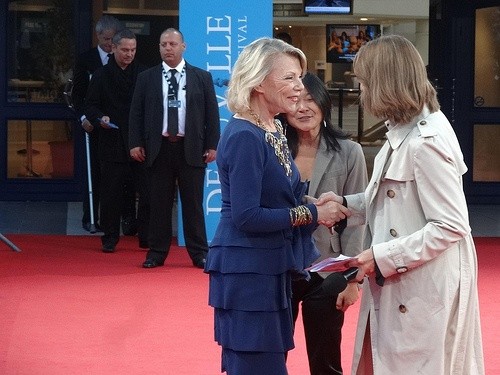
[276,32,292,45]
[340,32,352,54]
[72,14,125,232]
[316,36,485,375]
[204,36,351,375]
[328,31,343,53]
[85,30,151,253]
[356,32,368,47]
[275,72,368,375]
[129,27,220,269]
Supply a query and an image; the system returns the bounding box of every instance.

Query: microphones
[320,266,359,297]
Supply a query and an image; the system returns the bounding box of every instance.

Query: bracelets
[290,206,312,226]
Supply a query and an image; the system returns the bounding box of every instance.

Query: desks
[328,88,359,128]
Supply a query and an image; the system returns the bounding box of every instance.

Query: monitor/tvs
[325,23,382,64]
[303,0,353,14]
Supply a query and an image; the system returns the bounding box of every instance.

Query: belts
[162,139,185,146]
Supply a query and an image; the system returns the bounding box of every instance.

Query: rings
[131,155,135,157]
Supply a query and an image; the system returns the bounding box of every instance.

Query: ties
[107,52,112,64]
[167,69,178,137]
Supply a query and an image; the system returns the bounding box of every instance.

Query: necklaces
[247,105,292,177]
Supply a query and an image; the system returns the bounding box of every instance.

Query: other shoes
[83,222,101,232]
[194,258,206,269]
[143,262,163,267]
[103,247,114,253]
